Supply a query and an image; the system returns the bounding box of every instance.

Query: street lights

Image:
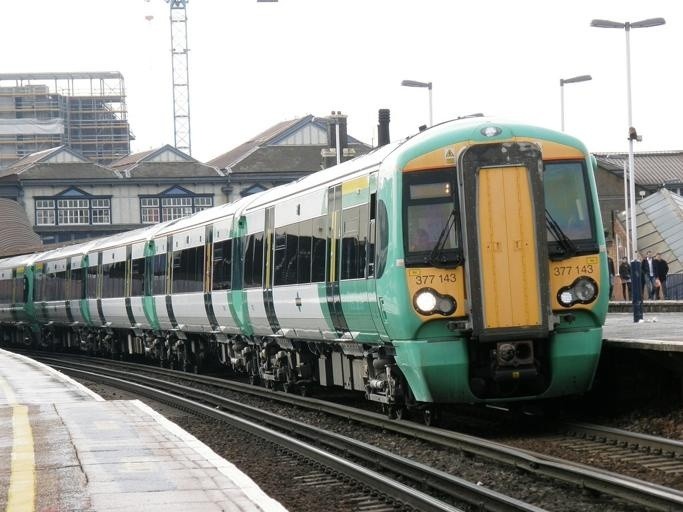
[557,74,592,133]
[329,110,343,164]
[590,16,669,263]
[606,153,631,267]
[399,79,435,127]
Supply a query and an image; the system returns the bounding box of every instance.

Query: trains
[0,118,615,426]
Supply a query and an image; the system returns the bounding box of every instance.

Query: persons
[606,249,669,300]
